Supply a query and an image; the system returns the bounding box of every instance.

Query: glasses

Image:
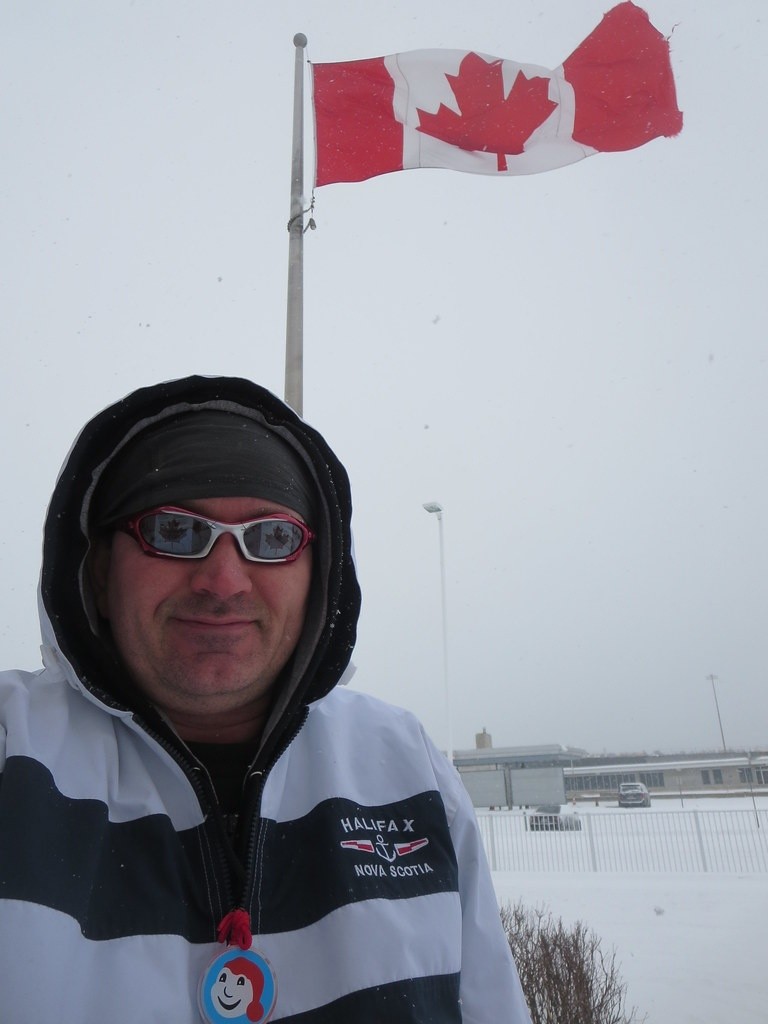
[115,505,319,565]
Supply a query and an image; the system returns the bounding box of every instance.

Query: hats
[88,406,322,541]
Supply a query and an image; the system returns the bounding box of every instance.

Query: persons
[0,375,534,1024]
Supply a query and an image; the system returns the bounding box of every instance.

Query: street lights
[423,501,454,751]
[708,673,727,753]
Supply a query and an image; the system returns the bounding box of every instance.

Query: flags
[308,0,685,189]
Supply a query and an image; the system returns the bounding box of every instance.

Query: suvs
[618,781,652,808]
